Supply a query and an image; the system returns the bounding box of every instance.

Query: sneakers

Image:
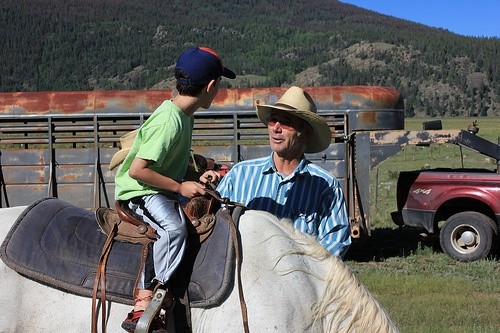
[120,308,169,333]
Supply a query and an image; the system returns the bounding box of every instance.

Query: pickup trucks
[389,167,500,261]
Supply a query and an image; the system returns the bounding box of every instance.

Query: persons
[114,45,237,333]
[212,86,352,257]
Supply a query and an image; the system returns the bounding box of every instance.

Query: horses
[0,196,401,333]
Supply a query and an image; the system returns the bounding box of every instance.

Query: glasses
[266,116,298,129]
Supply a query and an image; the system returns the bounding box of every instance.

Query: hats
[174,46,236,86]
[254,85,332,154]
